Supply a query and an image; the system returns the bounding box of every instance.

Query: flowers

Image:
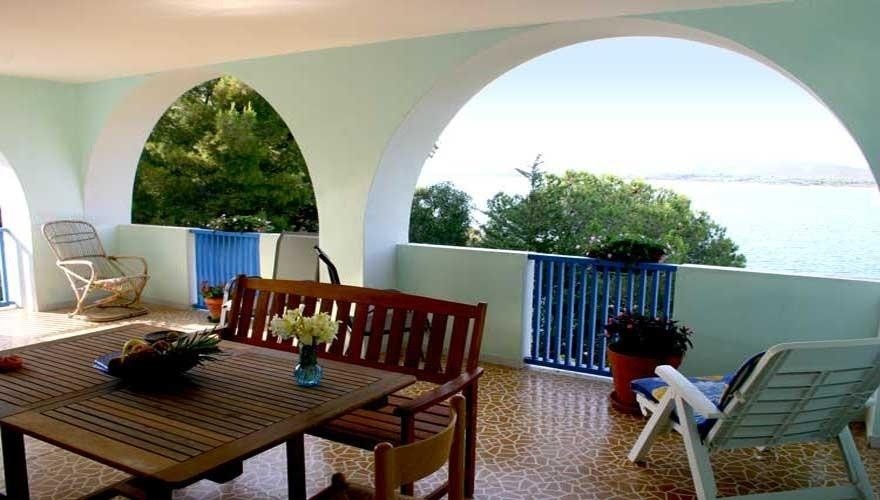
[267,303,343,344]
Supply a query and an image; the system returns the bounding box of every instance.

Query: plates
[93,350,192,378]
[145,330,186,345]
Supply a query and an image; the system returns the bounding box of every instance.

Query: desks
[0,323,416,499]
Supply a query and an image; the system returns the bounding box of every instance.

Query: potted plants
[602,307,697,414]
[196,281,225,323]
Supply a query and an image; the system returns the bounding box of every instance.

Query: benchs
[191,273,489,500]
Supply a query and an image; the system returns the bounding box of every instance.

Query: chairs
[303,365,485,500]
[41,219,151,321]
[627,337,880,500]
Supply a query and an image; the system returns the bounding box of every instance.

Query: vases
[294,347,323,387]
